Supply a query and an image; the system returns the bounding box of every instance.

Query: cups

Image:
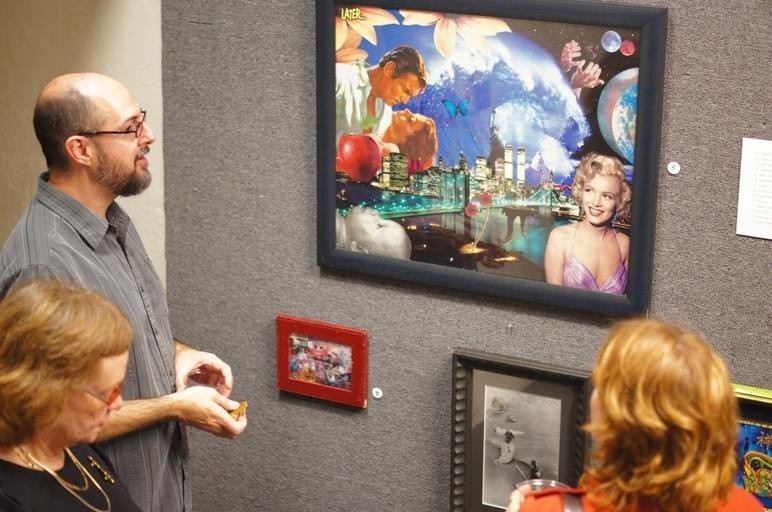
[186,368,225,393]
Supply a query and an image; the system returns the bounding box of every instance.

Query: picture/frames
[447,348,593,510]
[315,0,668,319]
[274,316,368,409]
[725,379,772,511]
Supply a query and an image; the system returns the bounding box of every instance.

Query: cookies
[228,399,248,418]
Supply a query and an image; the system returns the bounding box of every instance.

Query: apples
[340,132,381,182]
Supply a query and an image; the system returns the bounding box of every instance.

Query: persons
[560,36,605,97]
[0,73,248,511]
[334,205,413,262]
[506,318,767,512]
[334,44,437,173]
[1,276,143,511]
[544,150,629,296]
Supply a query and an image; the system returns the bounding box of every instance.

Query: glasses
[75,111,146,138]
[83,381,122,406]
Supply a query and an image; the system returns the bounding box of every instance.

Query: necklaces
[13,441,111,511]
